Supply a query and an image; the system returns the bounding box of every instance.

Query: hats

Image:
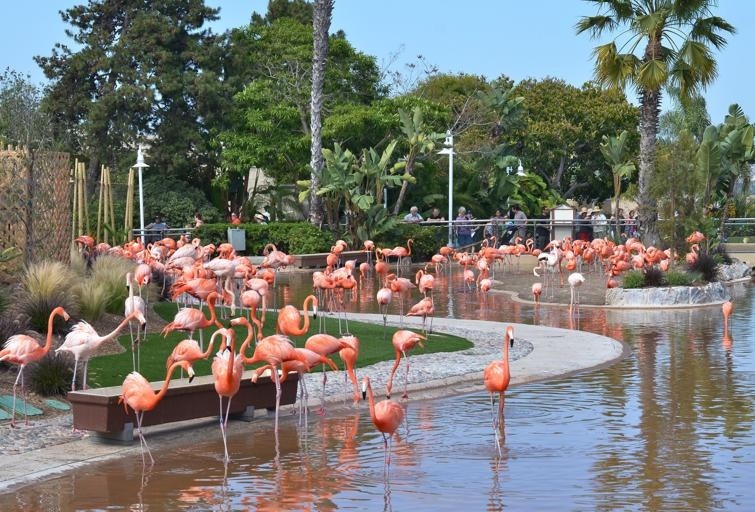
[580,206,601,216]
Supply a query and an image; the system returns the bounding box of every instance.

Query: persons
[144,215,168,243]
[404,205,640,252]
[193,212,204,227]
[253,213,267,225]
[231,211,241,225]
[184,224,192,241]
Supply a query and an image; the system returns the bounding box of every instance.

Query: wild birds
[72,232,454,340]
[722,301,733,326]
[360,379,405,466]
[1,306,70,430]
[485,326,515,448]
[165,328,232,379]
[211,328,244,462]
[253,347,338,415]
[303,332,344,393]
[119,361,196,464]
[124,271,149,374]
[722,326,733,349]
[56,310,148,391]
[384,327,427,404]
[455,234,706,313]
[228,316,301,432]
[338,334,363,383]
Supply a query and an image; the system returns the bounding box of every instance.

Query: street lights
[506,158,525,219]
[437,130,458,249]
[132,147,151,248]
